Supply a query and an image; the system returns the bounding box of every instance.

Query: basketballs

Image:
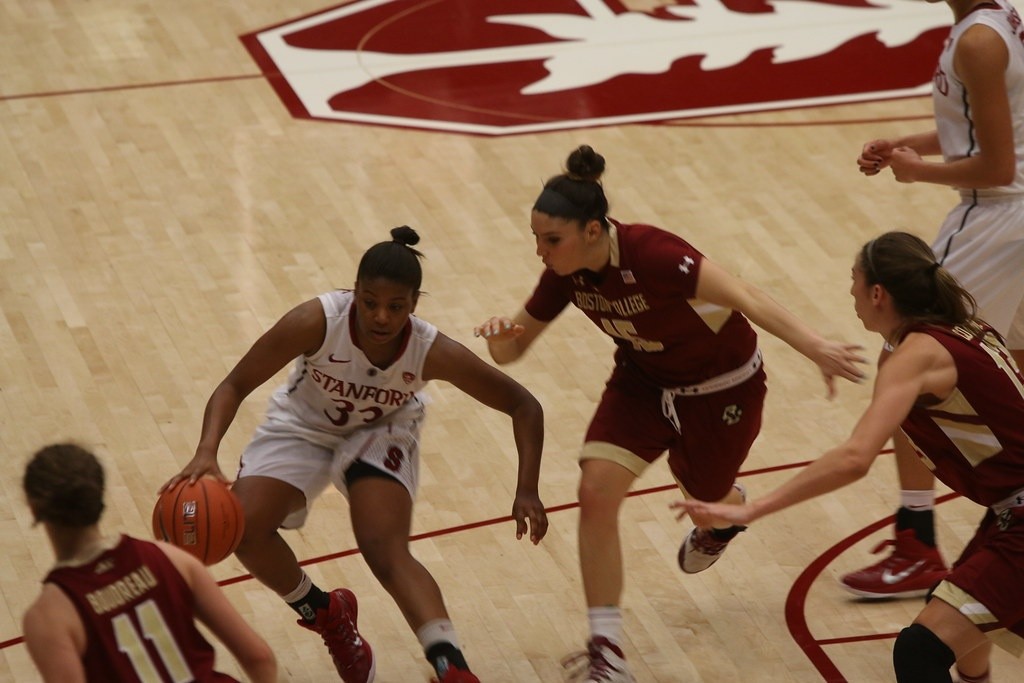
[153,478,244,567]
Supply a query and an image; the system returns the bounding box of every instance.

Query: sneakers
[297,588,376,683]
[840,529,950,599]
[677,480,746,573]
[580,651,635,683]
[430,665,480,683]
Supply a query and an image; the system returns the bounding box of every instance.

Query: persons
[838,1,1024,598]
[22,442,278,683]
[473,145,872,682]
[156,225,549,683]
[669,230,1024,683]
